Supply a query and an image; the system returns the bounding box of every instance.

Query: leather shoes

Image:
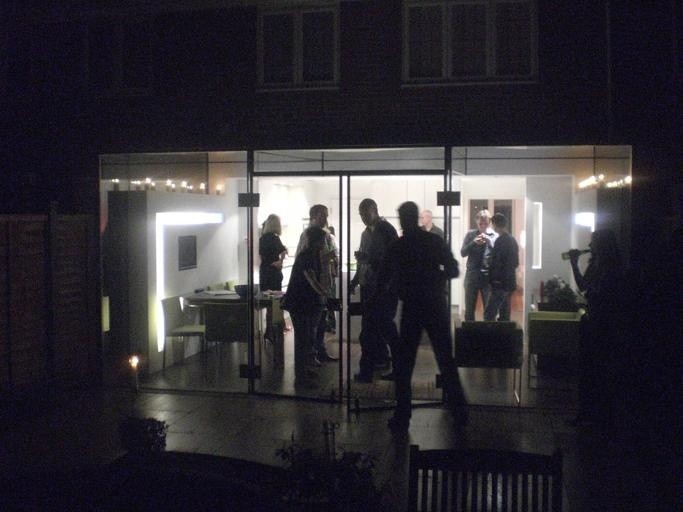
[297,352,338,376]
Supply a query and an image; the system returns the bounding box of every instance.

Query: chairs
[404,443,563,511]
[159,279,284,379]
[455,321,524,404]
[526,307,586,392]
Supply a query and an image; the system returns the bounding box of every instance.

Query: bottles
[561,249,592,260]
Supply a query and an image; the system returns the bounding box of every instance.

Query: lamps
[127,354,140,396]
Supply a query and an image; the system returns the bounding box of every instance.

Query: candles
[111,176,222,196]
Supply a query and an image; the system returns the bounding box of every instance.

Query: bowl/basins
[234,284,258,299]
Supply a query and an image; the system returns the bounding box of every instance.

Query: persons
[384,201,473,429]
[258,213,290,335]
[459,209,499,321]
[417,209,444,239]
[568,229,624,414]
[279,204,337,379]
[349,198,399,383]
[321,220,338,335]
[477,212,519,320]
